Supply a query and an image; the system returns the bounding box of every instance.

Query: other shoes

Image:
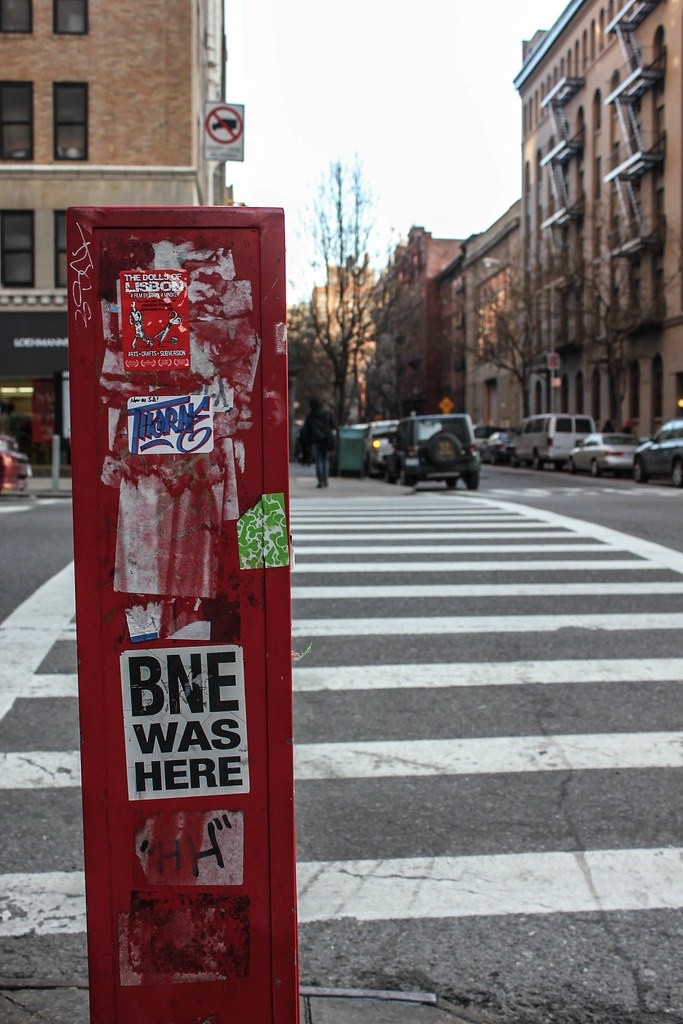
[317,481,327,488]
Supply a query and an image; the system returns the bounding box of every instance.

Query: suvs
[391,413,482,492]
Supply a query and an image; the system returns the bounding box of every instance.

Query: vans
[509,411,599,471]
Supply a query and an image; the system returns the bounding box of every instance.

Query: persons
[302,397,338,486]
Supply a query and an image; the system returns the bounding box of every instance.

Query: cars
[566,431,644,478]
[479,429,514,466]
[631,416,683,489]
[0,433,34,496]
[350,418,503,479]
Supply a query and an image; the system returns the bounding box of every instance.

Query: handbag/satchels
[323,413,339,451]
